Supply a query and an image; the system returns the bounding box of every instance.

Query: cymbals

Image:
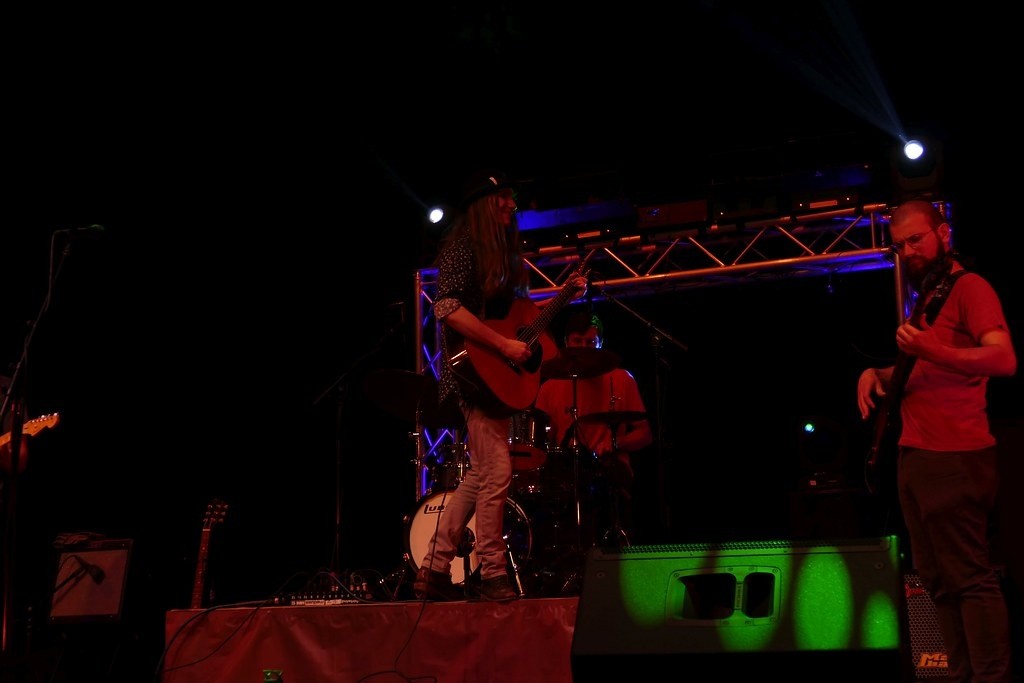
[540,346,623,381]
[580,410,657,423]
[360,367,457,427]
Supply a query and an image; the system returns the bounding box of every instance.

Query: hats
[462,170,514,208]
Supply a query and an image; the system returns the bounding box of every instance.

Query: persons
[413,168,588,602]
[857,198,1018,683]
[533,309,652,546]
[0,365,29,669]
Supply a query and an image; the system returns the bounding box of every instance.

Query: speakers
[903,565,1009,683]
[48,538,134,625]
[570,534,915,683]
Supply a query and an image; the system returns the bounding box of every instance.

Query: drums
[524,448,624,556]
[403,485,533,588]
[506,407,553,473]
[435,442,470,489]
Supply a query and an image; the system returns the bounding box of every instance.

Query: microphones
[54,224,105,238]
[75,556,104,581]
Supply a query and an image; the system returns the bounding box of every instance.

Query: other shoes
[468,569,517,603]
[415,567,455,601]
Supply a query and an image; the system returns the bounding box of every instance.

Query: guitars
[866,248,961,487]
[448,261,593,420]
[189,496,233,610]
[0,411,63,447]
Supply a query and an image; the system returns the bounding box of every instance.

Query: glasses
[890,227,935,252]
